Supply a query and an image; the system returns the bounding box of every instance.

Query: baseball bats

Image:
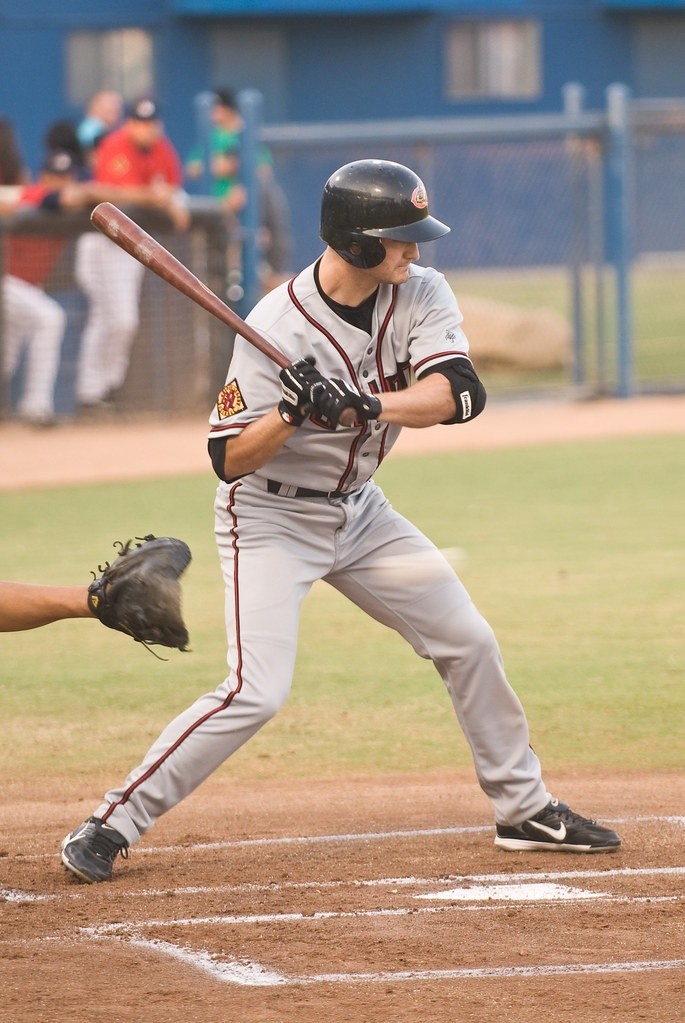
[88,201,358,425]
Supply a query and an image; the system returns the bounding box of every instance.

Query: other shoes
[82,393,130,416]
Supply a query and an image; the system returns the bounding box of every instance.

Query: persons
[0,534,193,661]
[61,159,621,884]
[0,85,298,429]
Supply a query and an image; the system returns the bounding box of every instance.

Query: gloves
[277,355,381,430]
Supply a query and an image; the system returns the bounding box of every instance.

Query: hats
[42,147,76,173]
[129,97,162,123]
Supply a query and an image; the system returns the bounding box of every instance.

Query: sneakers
[493,803,620,853]
[62,816,127,883]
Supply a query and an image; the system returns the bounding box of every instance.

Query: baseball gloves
[85,531,195,662]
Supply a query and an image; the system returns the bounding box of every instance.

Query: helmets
[319,158,452,270]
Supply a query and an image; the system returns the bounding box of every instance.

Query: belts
[269,478,342,499]
